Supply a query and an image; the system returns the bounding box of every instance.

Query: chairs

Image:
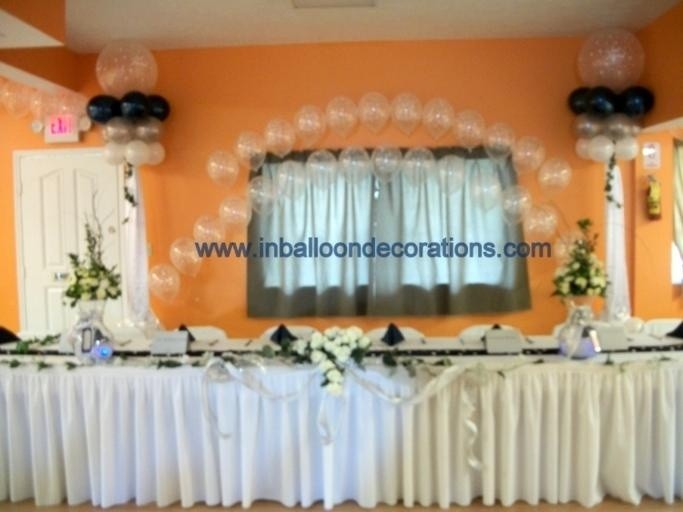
[364,326,424,341]
[257,324,320,343]
[151,326,228,343]
[457,324,525,343]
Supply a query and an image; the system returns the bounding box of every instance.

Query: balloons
[145,90,585,306]
[0,40,170,168]
[565,25,656,163]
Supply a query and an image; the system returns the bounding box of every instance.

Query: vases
[71,300,113,367]
[559,295,600,359]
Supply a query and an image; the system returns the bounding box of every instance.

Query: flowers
[546,215,615,300]
[295,325,370,396]
[49,218,123,307]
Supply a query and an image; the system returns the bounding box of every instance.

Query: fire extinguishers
[645,175,661,220]
